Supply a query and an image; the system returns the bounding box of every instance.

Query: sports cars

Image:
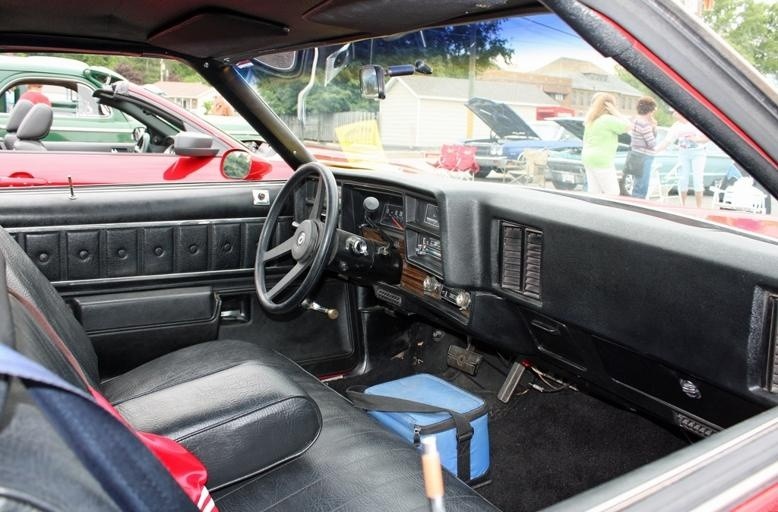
[0,53,446,188]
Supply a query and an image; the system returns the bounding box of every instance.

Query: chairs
[648,171,673,204]
[424,144,550,184]
[4,98,34,149]
[0,224,505,512]
[13,103,52,152]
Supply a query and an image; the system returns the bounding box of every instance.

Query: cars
[0,48,269,155]
[546,119,735,195]
[463,97,582,181]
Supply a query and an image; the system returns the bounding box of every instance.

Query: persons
[631,96,677,199]
[718,161,771,215]
[116,62,143,83]
[582,91,630,197]
[209,96,232,116]
[21,84,53,107]
[670,108,709,208]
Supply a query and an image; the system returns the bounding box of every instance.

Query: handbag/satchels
[624,151,644,175]
